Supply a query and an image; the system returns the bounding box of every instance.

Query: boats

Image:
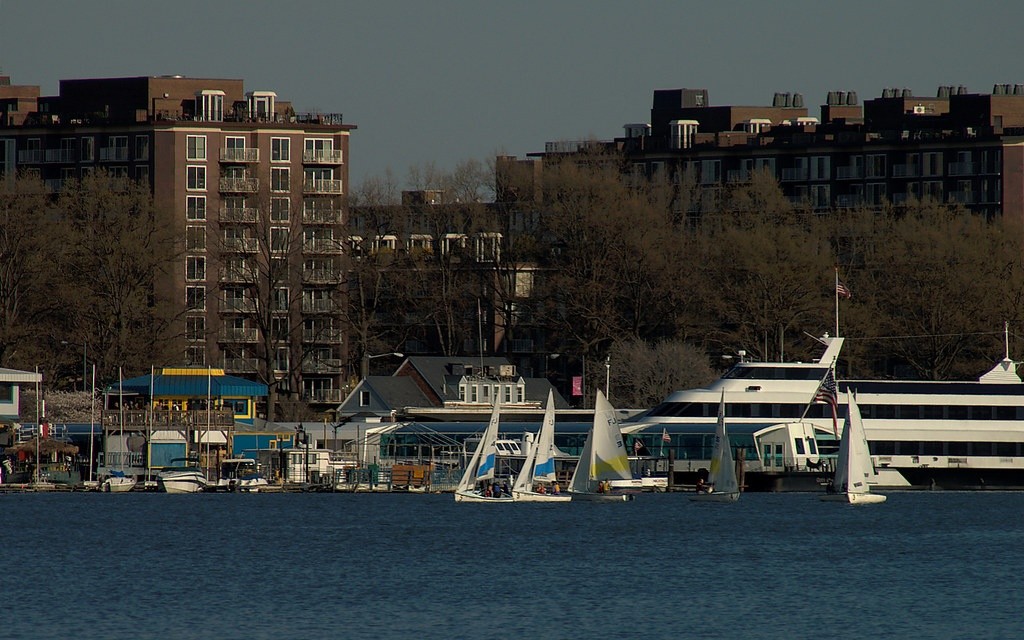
[216,457,268,493]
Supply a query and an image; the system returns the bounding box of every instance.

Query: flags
[809,366,838,432]
[838,280,851,299]
[664,430,671,443]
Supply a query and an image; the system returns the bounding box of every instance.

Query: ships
[636,266,1024,491]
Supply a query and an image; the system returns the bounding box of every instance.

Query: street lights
[61,340,87,391]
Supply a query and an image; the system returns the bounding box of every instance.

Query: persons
[479,480,509,498]
[598,478,612,492]
[535,482,547,493]
[551,481,560,495]
[114,400,179,425]
[696,479,710,495]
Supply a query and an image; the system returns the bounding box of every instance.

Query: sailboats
[452,384,514,503]
[567,388,670,501]
[687,387,741,503]
[32,366,211,494]
[819,385,888,505]
[510,387,573,503]
